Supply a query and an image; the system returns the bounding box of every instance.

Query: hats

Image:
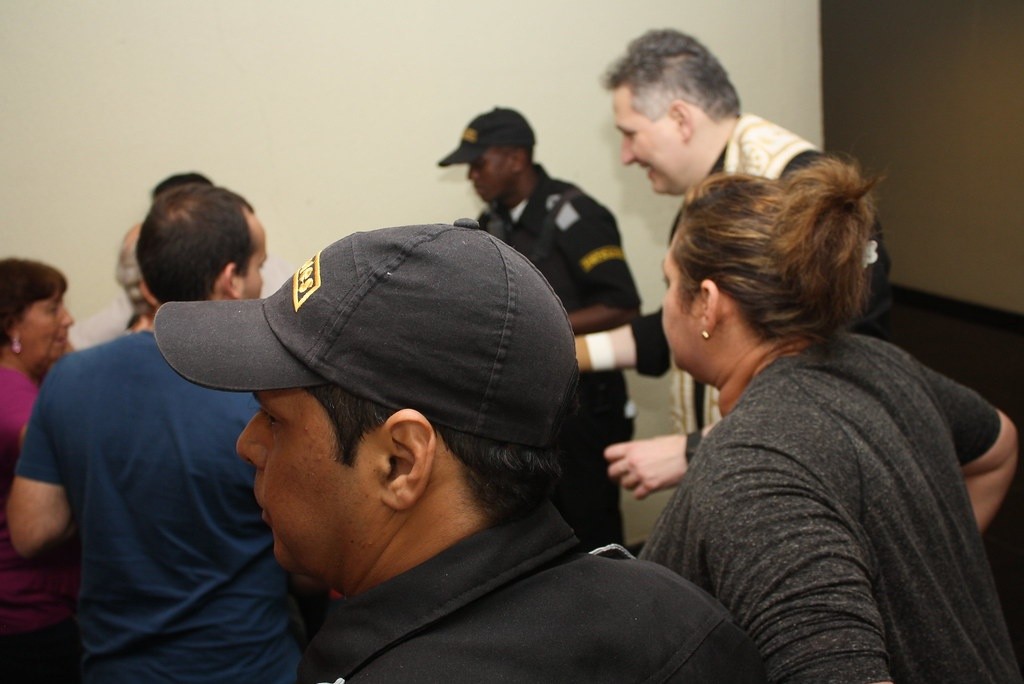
[438,109,536,166]
[152,218,576,449]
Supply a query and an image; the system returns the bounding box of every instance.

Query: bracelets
[585,332,615,372]
[685,432,701,460]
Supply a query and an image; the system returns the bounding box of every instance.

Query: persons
[0,31,1018,684]
